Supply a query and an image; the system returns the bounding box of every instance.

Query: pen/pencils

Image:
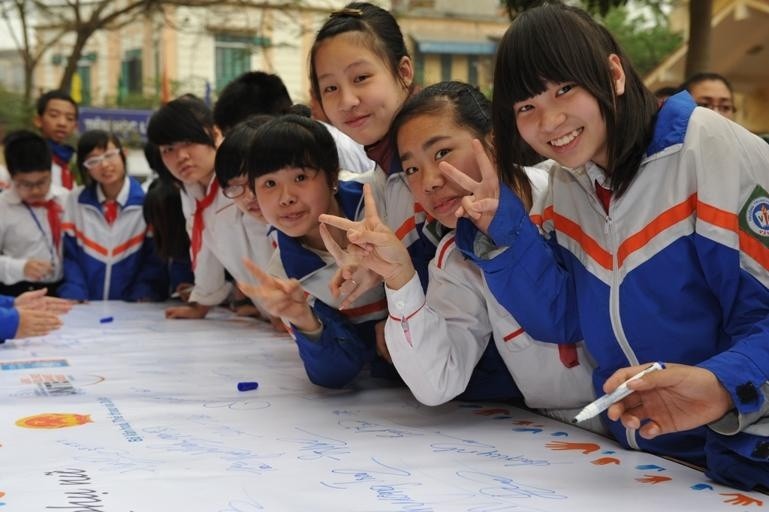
[572,361,666,424]
[171,286,193,298]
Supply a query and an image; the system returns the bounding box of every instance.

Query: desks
[0,300,769,512]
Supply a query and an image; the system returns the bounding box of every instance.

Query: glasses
[698,102,735,115]
[83,149,119,169]
[222,182,250,198]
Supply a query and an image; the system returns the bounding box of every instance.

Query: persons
[0,89,146,346]
[145,1,606,438]
[439,3,768,491]
[655,71,737,123]
